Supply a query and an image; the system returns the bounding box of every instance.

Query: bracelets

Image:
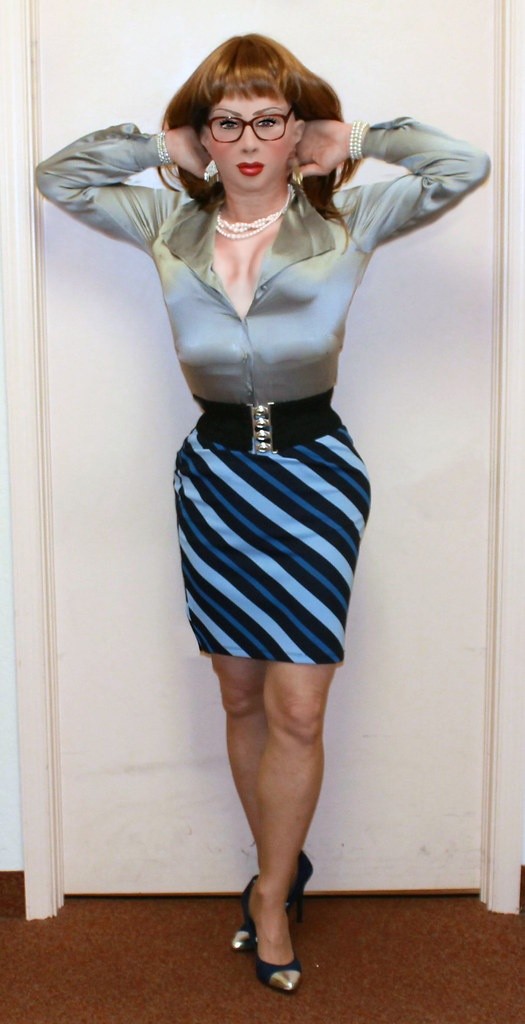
[348,119,369,161]
[155,129,173,166]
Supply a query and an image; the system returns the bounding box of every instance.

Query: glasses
[205,107,293,143]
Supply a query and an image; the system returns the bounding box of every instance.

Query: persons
[32,33,492,993]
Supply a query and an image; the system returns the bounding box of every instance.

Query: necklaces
[215,182,295,239]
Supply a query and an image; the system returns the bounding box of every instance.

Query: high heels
[241,875,301,989]
[231,850,313,951]
